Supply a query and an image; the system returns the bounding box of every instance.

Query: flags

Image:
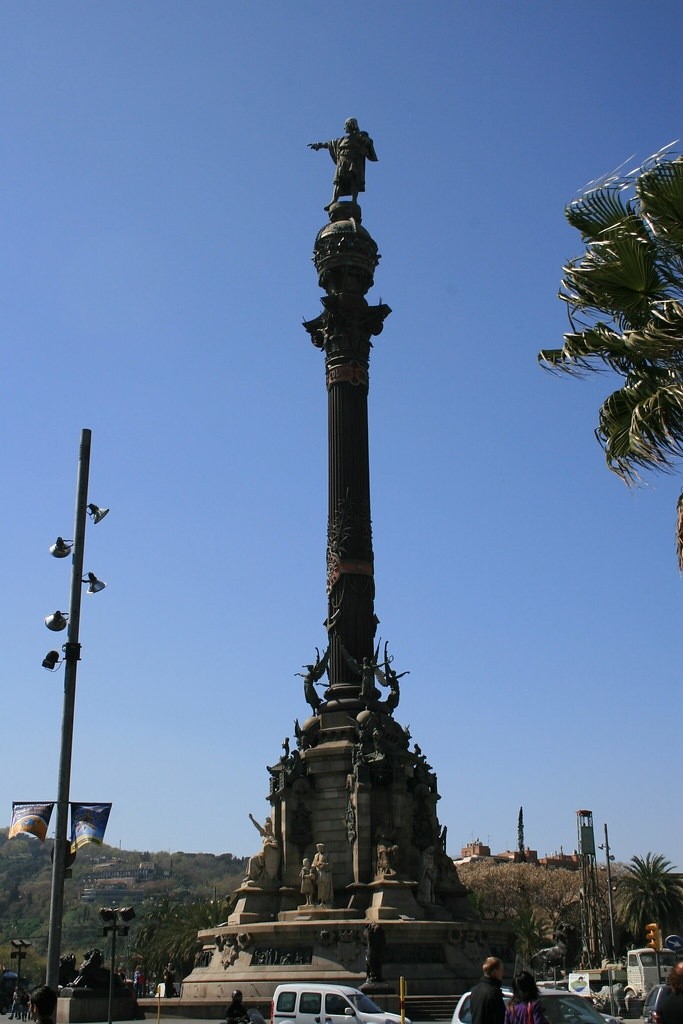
[70,801,112,854]
[8,801,54,843]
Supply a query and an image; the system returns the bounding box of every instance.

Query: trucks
[572,948,681,1019]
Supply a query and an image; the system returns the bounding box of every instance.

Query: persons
[470,957,506,1024]
[294,637,411,714]
[225,990,250,1024]
[307,118,374,211]
[309,843,334,906]
[117,963,177,998]
[243,813,280,883]
[300,859,315,905]
[504,971,551,1024]
[417,845,436,905]
[659,961,683,1024]
[8,986,57,1024]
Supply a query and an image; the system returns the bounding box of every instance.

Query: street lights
[11,939,32,988]
[100,906,135,1023]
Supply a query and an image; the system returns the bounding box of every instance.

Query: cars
[452,987,608,1024]
[270,984,411,1024]
[643,984,681,1024]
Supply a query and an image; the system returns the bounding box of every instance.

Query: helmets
[232,990,242,998]
